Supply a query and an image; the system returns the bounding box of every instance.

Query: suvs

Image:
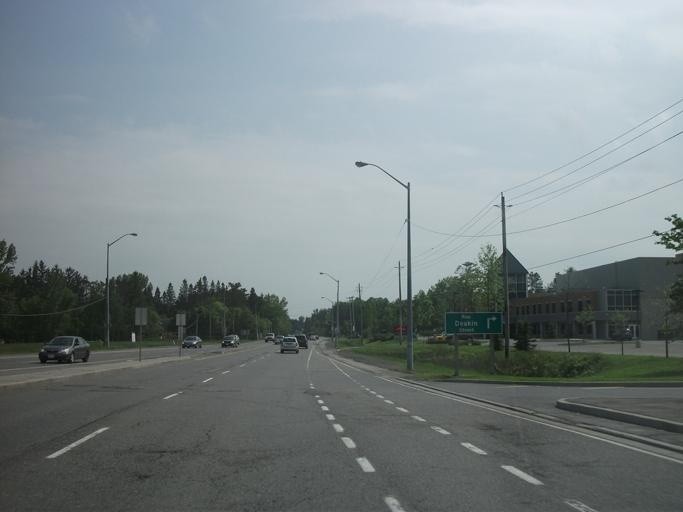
[612,328,632,340]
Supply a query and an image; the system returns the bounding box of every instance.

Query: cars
[426,330,475,346]
[182,334,203,349]
[36,335,91,363]
[275,334,284,344]
[279,336,299,353]
[295,334,308,349]
[231,334,239,345]
[220,335,238,349]
[263,332,274,343]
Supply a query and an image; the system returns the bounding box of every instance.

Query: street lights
[320,295,334,343]
[103,232,138,346]
[354,160,415,370]
[319,270,340,348]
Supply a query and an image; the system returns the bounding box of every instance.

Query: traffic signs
[444,311,503,334]
